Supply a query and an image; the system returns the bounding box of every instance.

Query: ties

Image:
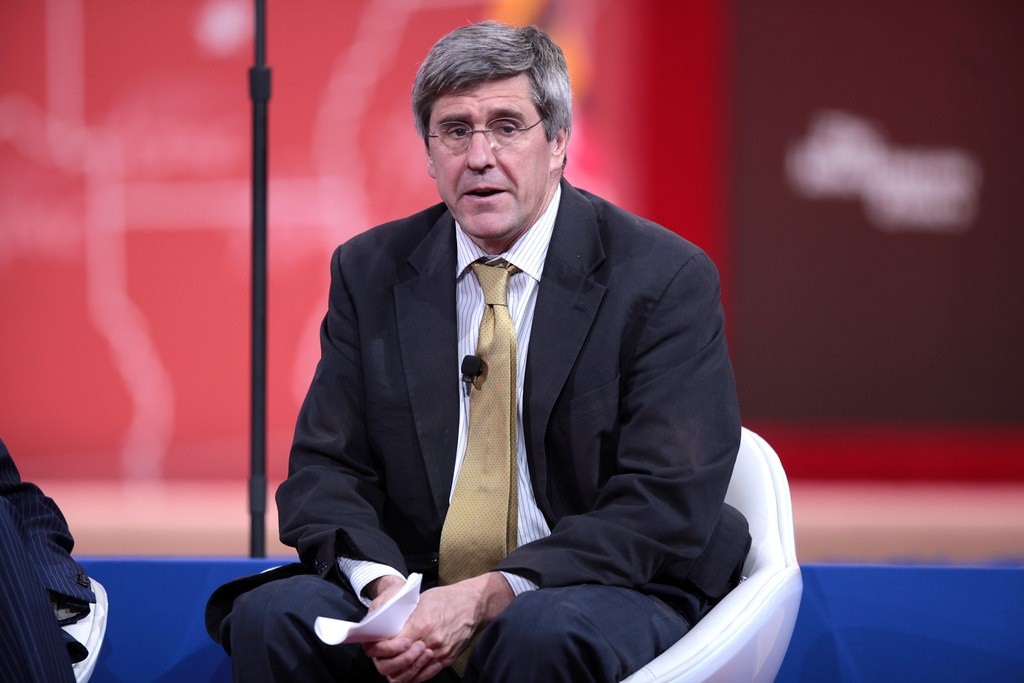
[438,260,519,680]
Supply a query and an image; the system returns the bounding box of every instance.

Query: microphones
[461,355,481,378]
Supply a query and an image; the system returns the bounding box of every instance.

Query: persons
[0,435,98,683]
[218,17,754,683]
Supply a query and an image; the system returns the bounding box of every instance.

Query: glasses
[424,116,546,152]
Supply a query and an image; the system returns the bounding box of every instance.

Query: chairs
[60,575,109,683]
[261,427,803,683]
